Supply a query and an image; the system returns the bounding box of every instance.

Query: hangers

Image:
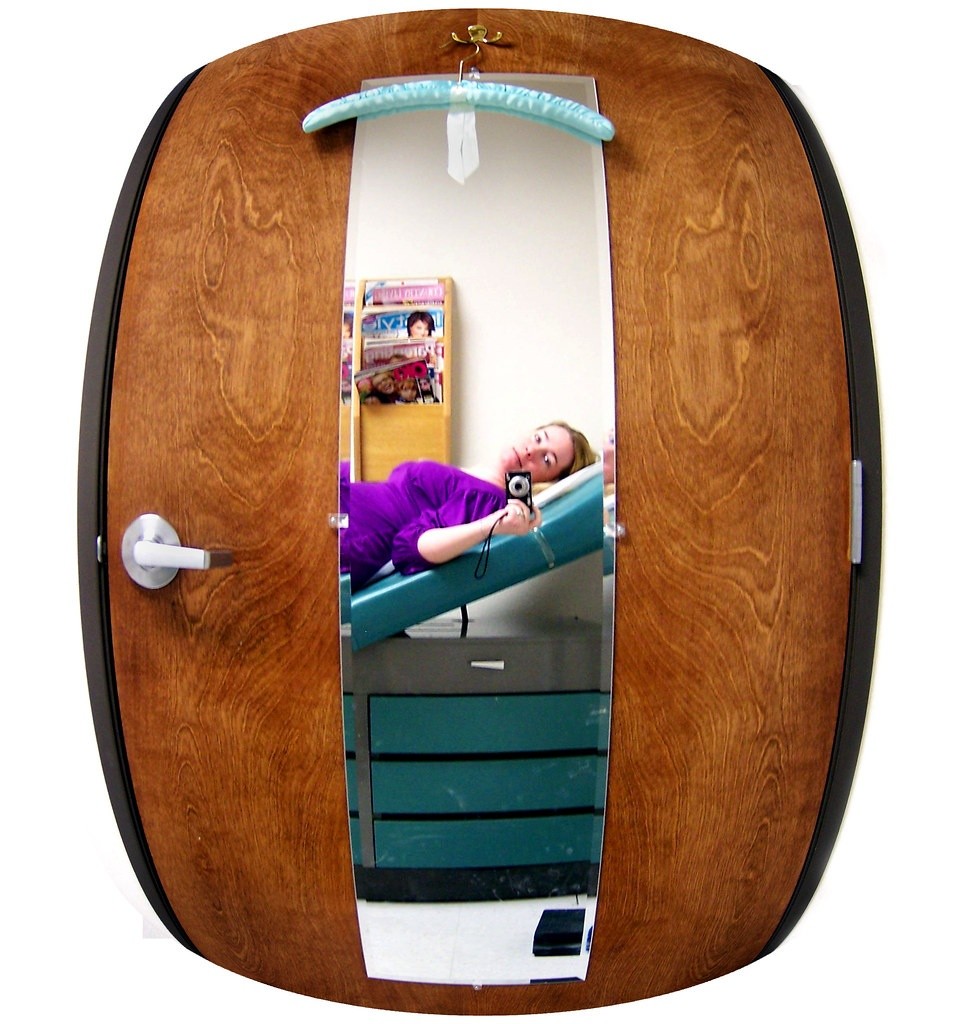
[303,36,615,143]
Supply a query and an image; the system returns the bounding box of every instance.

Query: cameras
[505,471,533,513]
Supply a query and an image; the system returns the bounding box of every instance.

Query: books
[341,280,446,397]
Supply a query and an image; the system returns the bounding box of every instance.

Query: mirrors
[339,73,618,988]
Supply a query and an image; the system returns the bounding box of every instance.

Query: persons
[407,310,435,338]
[339,422,596,596]
[357,372,436,404]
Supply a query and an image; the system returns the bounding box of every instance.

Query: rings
[516,510,524,518]
